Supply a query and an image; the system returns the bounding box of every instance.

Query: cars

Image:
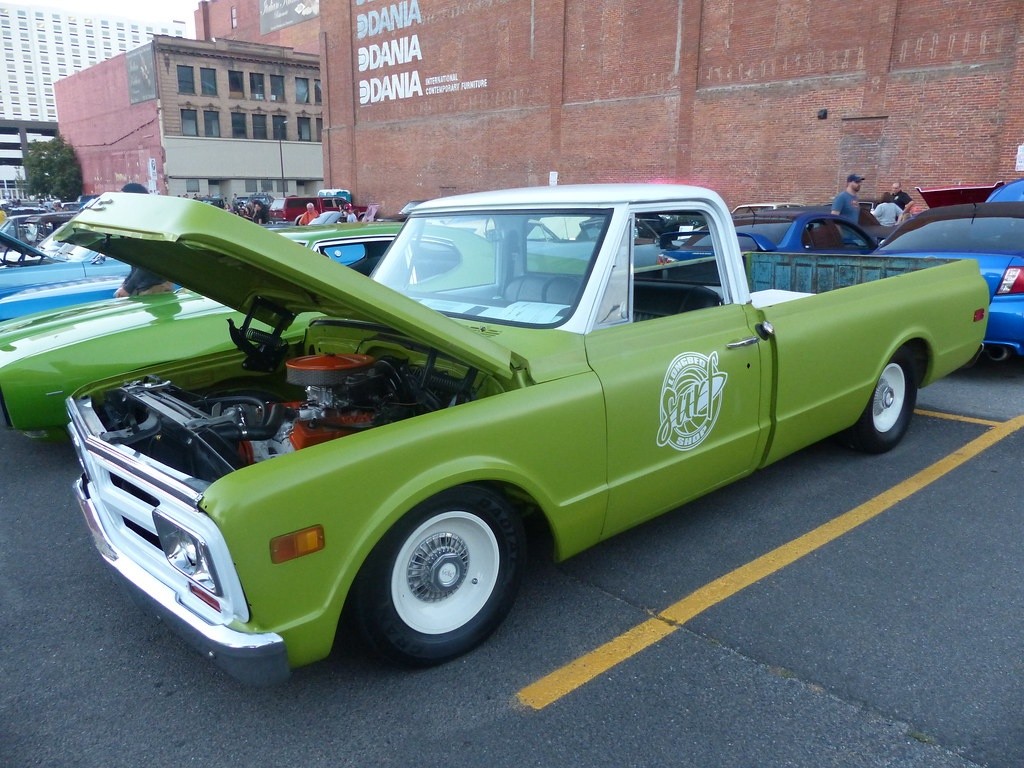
[308,210,366,225]
[872,179,1023,365]
[818,197,882,214]
[656,208,882,269]
[729,203,806,213]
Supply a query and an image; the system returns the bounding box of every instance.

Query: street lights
[278,121,289,199]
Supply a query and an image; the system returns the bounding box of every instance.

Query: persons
[830,174,866,247]
[347,210,358,223]
[2,192,78,238]
[299,203,320,226]
[339,206,344,213]
[253,203,266,224]
[148,191,249,222]
[891,182,914,221]
[870,191,904,245]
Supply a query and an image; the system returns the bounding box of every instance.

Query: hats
[847,174,865,182]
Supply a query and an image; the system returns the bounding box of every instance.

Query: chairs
[505,274,573,306]
[634,283,721,321]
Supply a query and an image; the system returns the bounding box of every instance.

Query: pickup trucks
[268,196,368,226]
[0,225,591,450]
[0,184,282,324]
[49,181,991,681]
